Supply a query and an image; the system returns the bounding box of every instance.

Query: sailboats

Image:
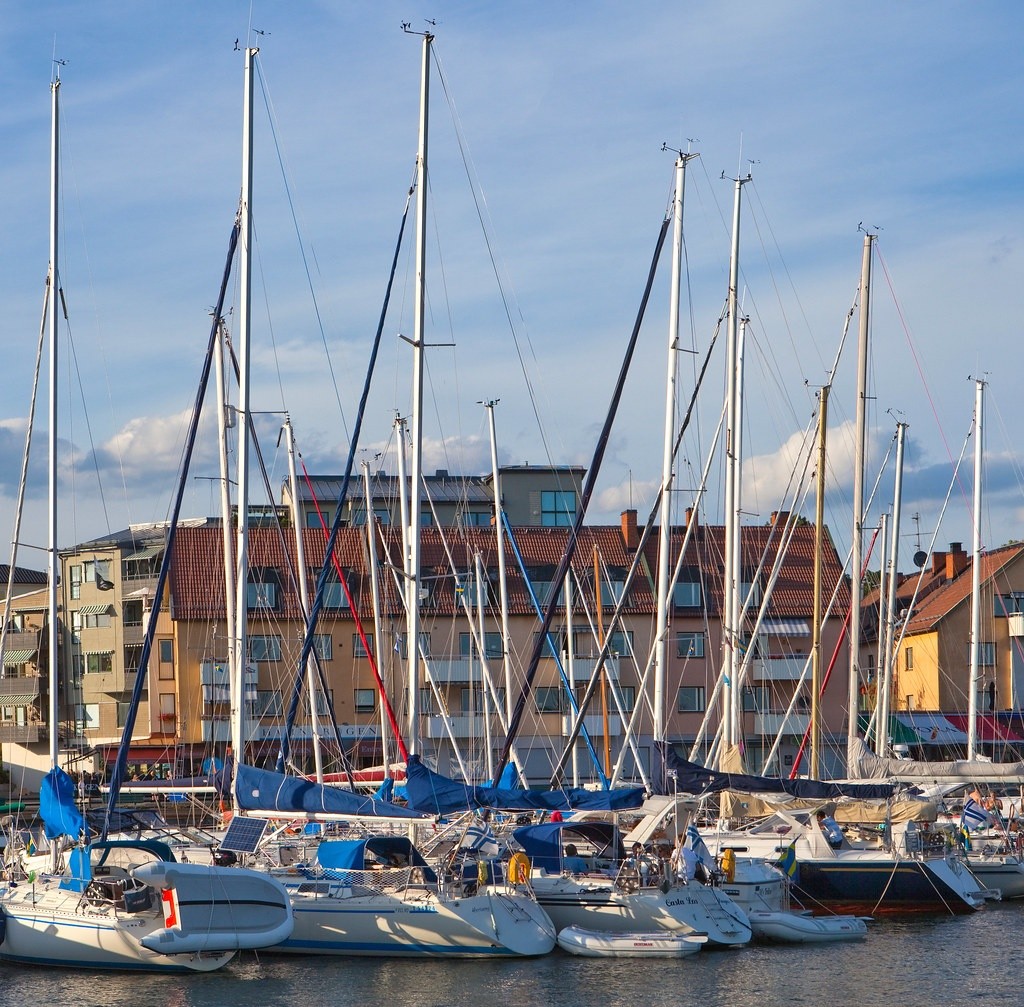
[0,0,1023,978]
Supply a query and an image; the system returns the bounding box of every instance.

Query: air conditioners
[33,662,40,667]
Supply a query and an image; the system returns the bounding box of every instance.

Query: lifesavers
[550,810,563,822]
[479,860,488,882]
[509,853,530,884]
[220,800,225,810]
[219,811,232,822]
[723,849,736,883]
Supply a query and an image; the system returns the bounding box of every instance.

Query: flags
[393,634,402,654]
[688,639,695,655]
[776,842,797,880]
[94,555,115,591]
[683,825,716,870]
[961,790,992,832]
[959,825,973,851]
[29,834,37,857]
[419,588,429,600]
[215,664,224,673]
[725,635,747,654]
[460,816,499,855]
[455,584,464,595]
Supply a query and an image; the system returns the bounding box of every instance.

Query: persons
[985,792,1003,814]
[563,844,587,873]
[68,769,104,785]
[129,775,140,782]
[550,810,563,823]
[150,771,160,801]
[27,706,43,722]
[817,811,843,850]
[629,842,651,867]
[921,823,930,837]
[656,832,698,880]
